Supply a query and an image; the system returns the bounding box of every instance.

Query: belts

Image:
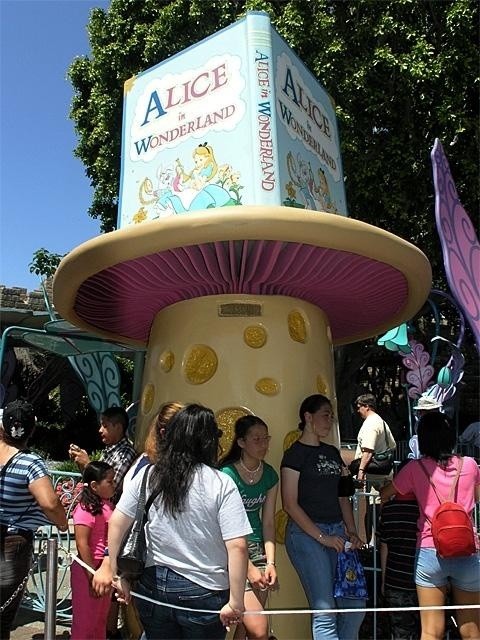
[0,526,32,536]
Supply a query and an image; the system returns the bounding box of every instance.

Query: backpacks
[416,457,476,559]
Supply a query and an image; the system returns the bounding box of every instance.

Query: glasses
[246,435,272,443]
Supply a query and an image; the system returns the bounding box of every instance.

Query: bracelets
[316,533,327,540]
[266,561,277,568]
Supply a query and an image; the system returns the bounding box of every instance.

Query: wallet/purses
[337,475,355,497]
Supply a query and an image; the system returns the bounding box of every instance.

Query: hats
[3,399,35,440]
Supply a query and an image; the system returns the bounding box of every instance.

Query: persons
[411,394,445,419]
[459,420,480,450]
[372,456,422,640]
[350,393,395,504]
[278,393,370,639]
[68,401,190,639]
[379,410,480,640]
[218,414,278,640]
[1,401,66,639]
[106,402,254,640]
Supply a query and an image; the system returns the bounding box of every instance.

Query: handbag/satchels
[331,548,370,600]
[348,421,394,475]
[117,463,154,575]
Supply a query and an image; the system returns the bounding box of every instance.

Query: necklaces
[241,455,262,484]
